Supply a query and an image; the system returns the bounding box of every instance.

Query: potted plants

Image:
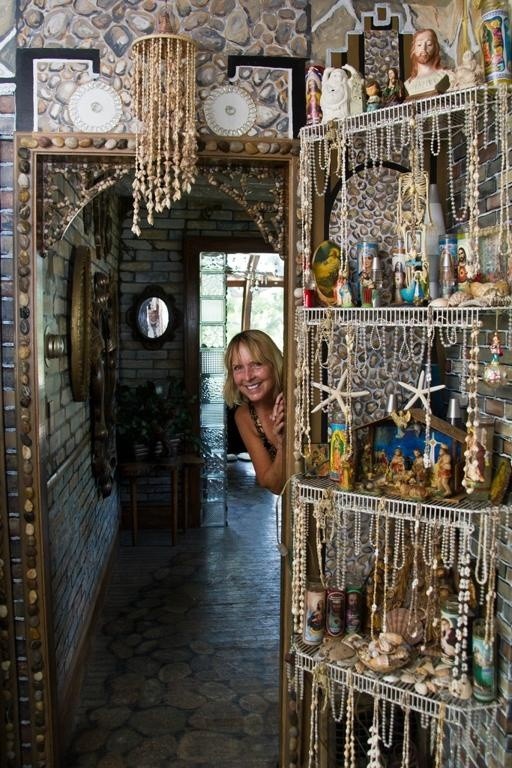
[117,383,210,463]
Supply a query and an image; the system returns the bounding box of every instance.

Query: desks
[119,453,204,547]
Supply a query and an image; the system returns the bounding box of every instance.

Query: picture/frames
[71,246,93,400]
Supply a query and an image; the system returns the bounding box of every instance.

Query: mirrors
[125,285,184,351]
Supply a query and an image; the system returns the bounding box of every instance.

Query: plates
[204,86,256,138]
[69,82,122,133]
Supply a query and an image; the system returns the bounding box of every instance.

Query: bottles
[445,398,461,429]
[369,257,383,308]
[303,254,317,308]
[439,255,452,299]
[471,620,500,702]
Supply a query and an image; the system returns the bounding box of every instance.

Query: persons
[222,329,285,495]
[306,77,321,122]
[381,67,404,107]
[364,80,380,112]
[360,435,453,501]
[402,28,450,96]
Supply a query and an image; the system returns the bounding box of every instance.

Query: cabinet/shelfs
[295,87,512,732]
[199,253,227,375]
[200,424,228,527]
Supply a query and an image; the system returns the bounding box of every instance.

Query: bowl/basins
[355,649,412,674]
